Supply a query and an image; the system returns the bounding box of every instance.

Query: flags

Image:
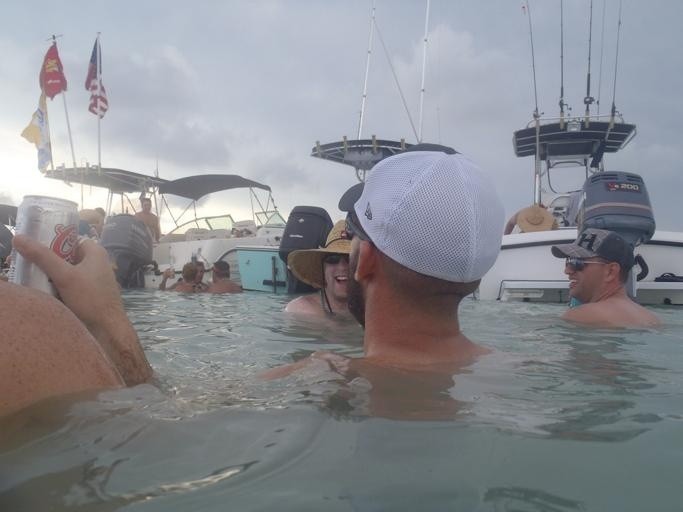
[20,100,53,171]
[38,44,65,99]
[84,42,110,120]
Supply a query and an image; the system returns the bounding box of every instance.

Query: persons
[79,207,106,239]
[549,227,661,330]
[159,261,244,293]
[0,234,154,420]
[250,152,506,381]
[506,203,559,233]
[282,218,363,319]
[71,235,89,259]
[133,200,160,241]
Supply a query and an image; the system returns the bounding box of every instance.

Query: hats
[287,220,353,288]
[338,151,505,283]
[552,228,635,271]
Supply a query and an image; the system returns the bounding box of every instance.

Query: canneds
[8,195,79,301]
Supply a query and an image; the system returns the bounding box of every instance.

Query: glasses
[566,257,606,271]
[345,212,372,247]
[322,255,350,264]
